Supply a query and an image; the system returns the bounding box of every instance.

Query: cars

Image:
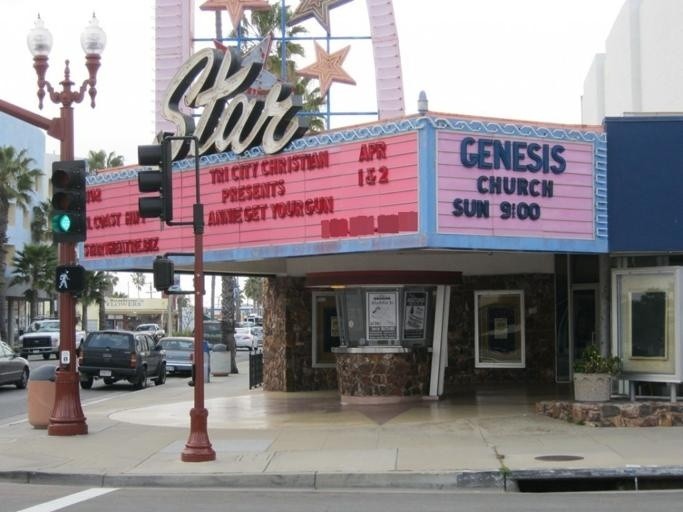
[135,322,194,374]
[202,313,264,353]
[0,337,29,390]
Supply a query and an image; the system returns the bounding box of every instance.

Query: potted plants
[571,345,624,403]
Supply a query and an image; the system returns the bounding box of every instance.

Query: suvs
[77,328,166,389]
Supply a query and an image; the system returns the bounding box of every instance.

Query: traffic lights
[136,128,173,223]
[51,159,86,243]
[153,256,175,291]
[53,264,86,293]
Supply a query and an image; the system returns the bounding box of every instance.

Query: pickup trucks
[17,316,87,358]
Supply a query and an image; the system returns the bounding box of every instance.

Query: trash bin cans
[27,365,61,430]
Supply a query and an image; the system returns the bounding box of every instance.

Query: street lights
[26,9,106,438]
